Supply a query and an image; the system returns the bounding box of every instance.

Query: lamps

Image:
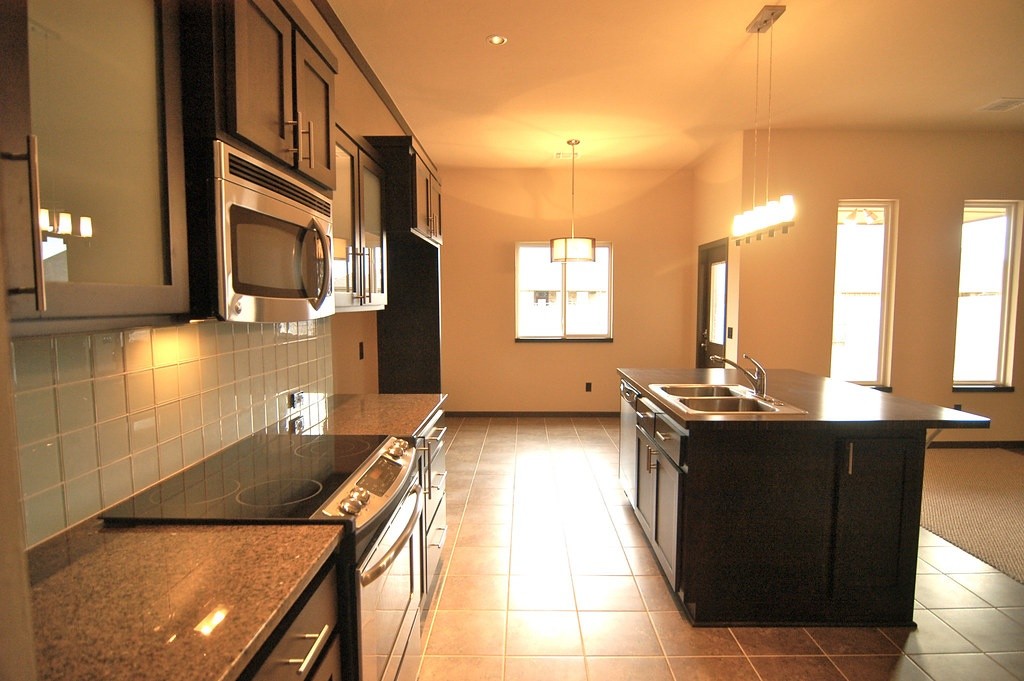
[729,5,797,246]
[863,208,879,224]
[550,139,596,262]
[846,209,858,227]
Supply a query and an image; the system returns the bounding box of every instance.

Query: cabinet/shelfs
[416,409,448,594]
[363,135,444,246]
[0,0,190,338]
[251,565,342,681]
[635,397,927,628]
[333,126,387,313]
[179,0,338,190]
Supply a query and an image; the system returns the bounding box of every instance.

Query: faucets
[710,354,766,398]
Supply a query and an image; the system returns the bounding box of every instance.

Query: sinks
[650,382,751,397]
[671,398,782,413]
